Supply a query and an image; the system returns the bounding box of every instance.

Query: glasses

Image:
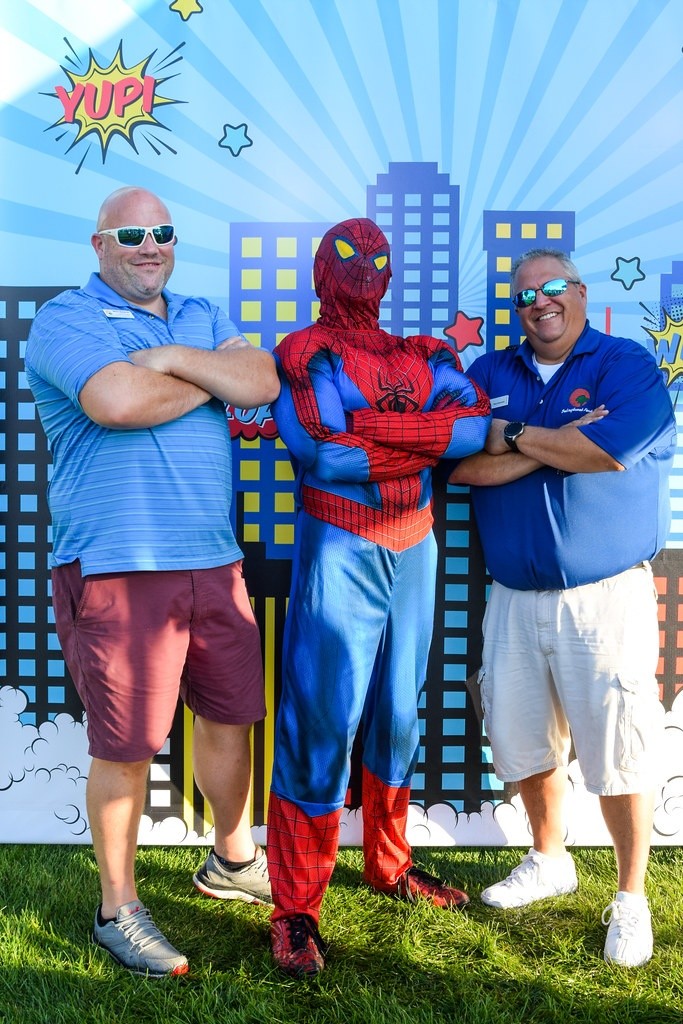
[512,279,580,308]
[99,224,174,248]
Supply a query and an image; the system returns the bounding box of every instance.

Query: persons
[447,248,677,969]
[25,187,281,974]
[266,218,493,977]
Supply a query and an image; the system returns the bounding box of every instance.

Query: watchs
[503,421,526,453]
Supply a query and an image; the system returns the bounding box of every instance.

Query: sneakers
[481,848,578,910]
[395,864,470,909]
[92,901,189,979]
[270,914,327,977]
[192,845,275,908]
[601,891,653,969]
